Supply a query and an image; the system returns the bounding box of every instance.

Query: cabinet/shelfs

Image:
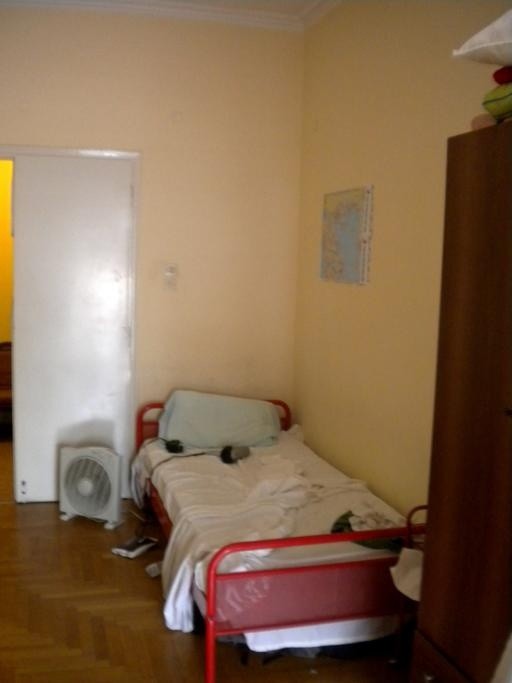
[415,128,510,682]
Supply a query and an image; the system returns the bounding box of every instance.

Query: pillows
[159,389,282,450]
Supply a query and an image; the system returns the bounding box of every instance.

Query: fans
[58,445,127,530]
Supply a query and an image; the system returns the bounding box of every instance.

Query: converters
[166,441,182,453]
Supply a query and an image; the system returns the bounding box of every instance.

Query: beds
[137,401,424,683]
